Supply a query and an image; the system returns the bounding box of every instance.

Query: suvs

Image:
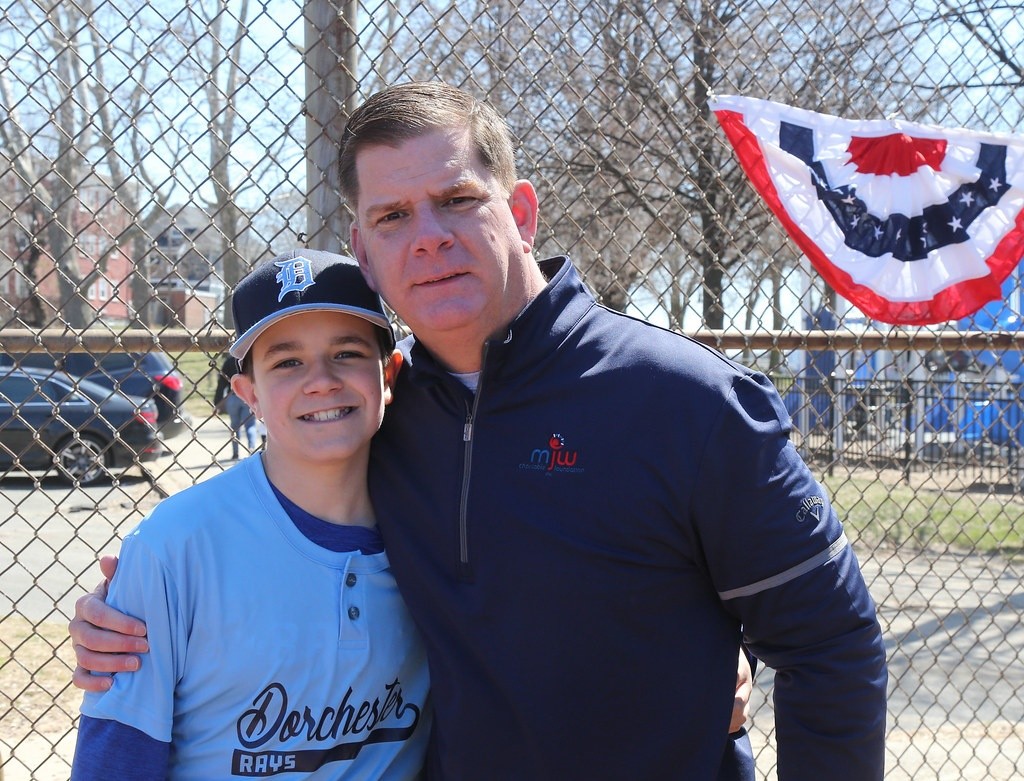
[0,352,194,440]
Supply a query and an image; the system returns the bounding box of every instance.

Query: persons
[70,250,756,781]
[69,81,888,781]
[213,359,257,460]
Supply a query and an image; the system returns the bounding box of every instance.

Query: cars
[0,367,162,486]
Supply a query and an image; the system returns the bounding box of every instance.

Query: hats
[227,249,397,371]
